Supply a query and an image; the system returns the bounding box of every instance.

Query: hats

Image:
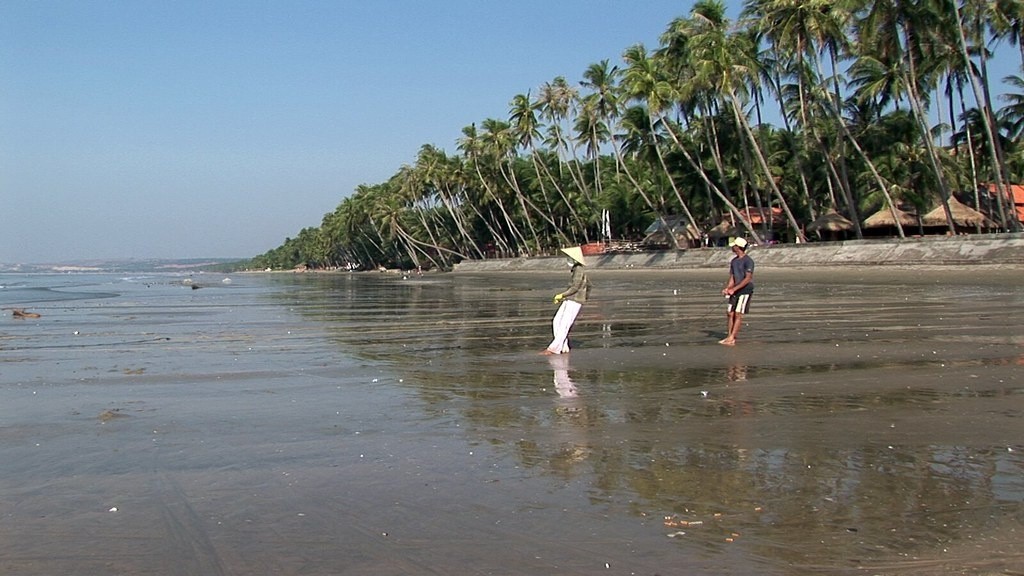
[560,246,587,267]
[729,236,748,250]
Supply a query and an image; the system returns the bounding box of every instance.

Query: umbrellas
[601,208,611,240]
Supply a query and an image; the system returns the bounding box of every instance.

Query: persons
[537,250,587,354]
[547,354,591,481]
[700,231,709,247]
[719,236,754,345]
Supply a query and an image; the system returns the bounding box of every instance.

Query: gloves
[553,300,559,304]
[555,294,562,299]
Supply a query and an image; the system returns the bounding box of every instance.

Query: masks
[567,257,576,267]
